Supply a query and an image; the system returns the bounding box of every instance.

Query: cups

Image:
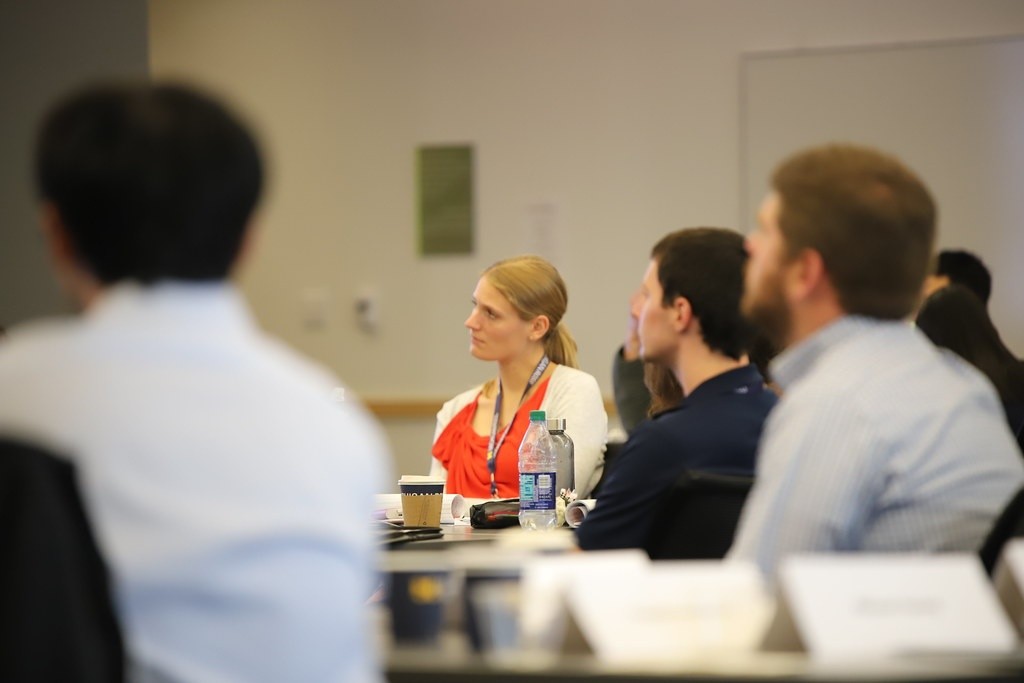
[397,474,446,534]
[379,561,563,669]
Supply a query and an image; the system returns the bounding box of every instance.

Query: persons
[429,255,608,500]
[569,228,780,562]
[0,78,390,683]
[914,251,1024,457]
[717,143,1024,578]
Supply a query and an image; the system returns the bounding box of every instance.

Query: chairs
[640,468,752,559]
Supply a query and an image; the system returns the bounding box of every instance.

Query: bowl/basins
[561,550,1018,673]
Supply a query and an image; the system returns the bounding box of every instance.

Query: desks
[382,525,560,626]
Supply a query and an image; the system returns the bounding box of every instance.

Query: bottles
[518,410,558,532]
[545,418,576,504]
